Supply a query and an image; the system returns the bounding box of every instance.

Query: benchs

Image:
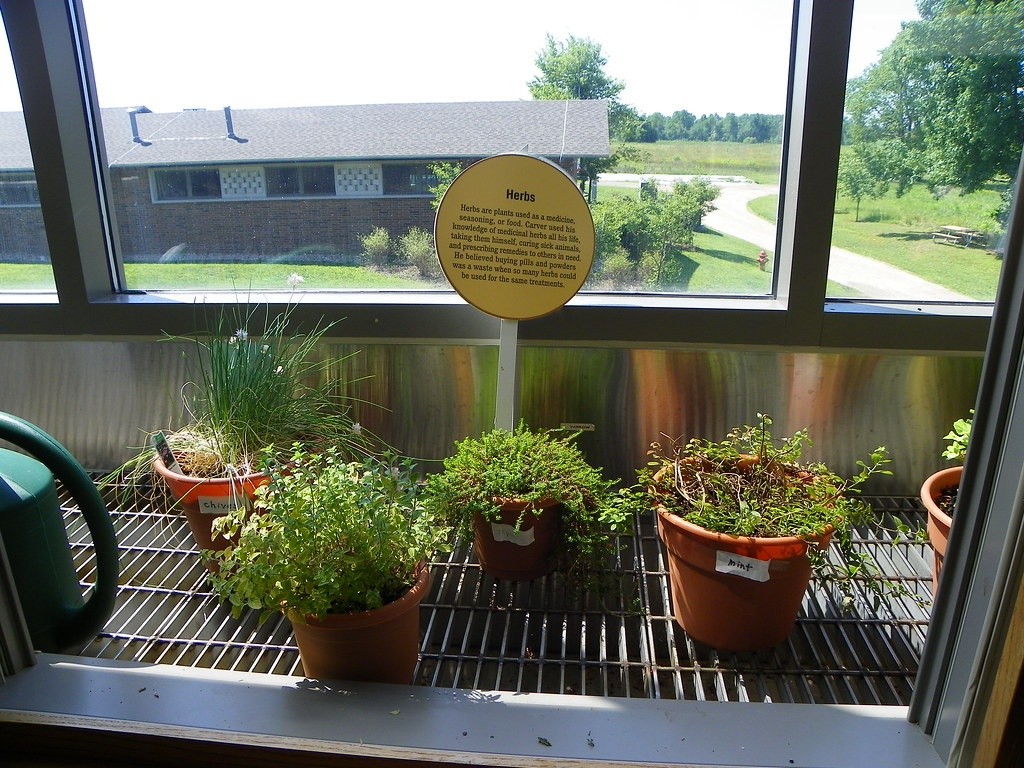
[932,232,962,244]
[955,231,984,245]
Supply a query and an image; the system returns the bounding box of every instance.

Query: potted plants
[922,408,975,602]
[622,411,929,650]
[200,439,455,686]
[425,420,635,615]
[94,276,450,576]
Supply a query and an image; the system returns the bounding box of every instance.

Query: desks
[940,225,979,246]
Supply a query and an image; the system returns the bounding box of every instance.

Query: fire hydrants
[756,250,770,271]
